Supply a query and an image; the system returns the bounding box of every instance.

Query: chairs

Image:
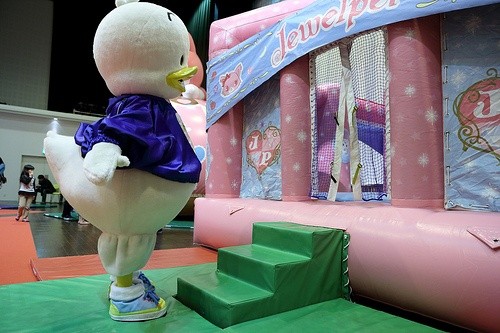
[50,184,61,203]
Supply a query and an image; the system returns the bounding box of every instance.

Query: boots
[22,208,30,222]
[78,216,89,225]
[15,206,25,221]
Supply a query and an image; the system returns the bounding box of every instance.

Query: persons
[62,200,74,220]
[78,215,90,225]
[31,175,56,204]
[15,165,36,222]
[0,156,5,210]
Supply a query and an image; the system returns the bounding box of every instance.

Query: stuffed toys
[43,0,206,322]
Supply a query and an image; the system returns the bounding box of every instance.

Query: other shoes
[62,214,74,220]
[31,201,36,204]
[40,201,46,204]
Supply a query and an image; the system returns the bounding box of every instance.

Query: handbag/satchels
[2,177,7,184]
